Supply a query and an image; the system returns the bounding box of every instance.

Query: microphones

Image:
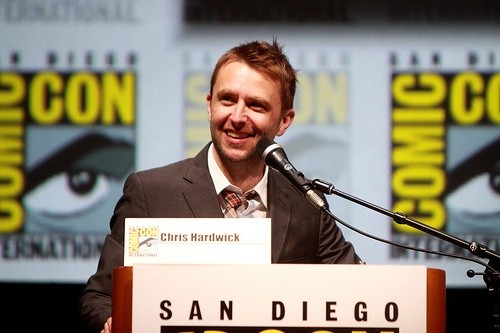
[256,137,327,211]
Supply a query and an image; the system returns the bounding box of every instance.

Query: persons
[79,35,366,333]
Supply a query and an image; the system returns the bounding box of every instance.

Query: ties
[221,189,255,218]
